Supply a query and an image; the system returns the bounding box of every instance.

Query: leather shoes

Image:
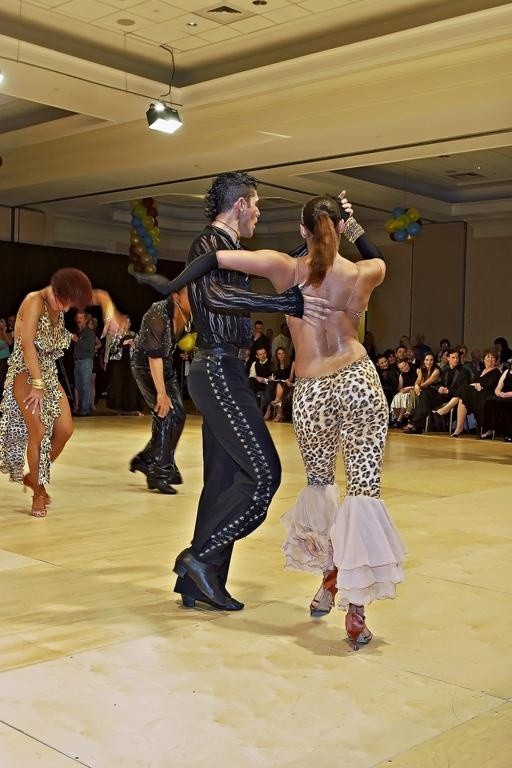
[147,476,176,495]
[264,405,272,420]
[129,455,149,475]
[180,593,245,611]
[172,548,234,607]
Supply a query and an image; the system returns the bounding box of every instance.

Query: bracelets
[103,317,113,321]
[29,377,46,389]
[340,216,364,242]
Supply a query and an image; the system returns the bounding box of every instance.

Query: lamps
[146,46,184,134]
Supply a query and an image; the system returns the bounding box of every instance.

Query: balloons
[387,205,423,244]
[127,197,162,275]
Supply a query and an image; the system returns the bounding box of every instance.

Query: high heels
[31,494,46,517]
[272,414,284,422]
[431,407,448,419]
[481,430,496,439]
[271,400,282,408]
[450,427,465,438]
[309,570,340,617]
[23,475,50,505]
[346,612,373,651]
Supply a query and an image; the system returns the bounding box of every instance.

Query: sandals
[403,423,415,430]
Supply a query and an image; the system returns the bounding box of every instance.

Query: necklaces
[175,304,193,332]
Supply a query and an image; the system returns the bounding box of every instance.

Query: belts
[200,344,245,359]
[73,357,84,360]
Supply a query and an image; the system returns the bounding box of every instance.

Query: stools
[426,400,470,436]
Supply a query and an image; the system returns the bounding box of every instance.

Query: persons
[171,171,355,616]
[1,268,130,516]
[0,311,510,441]
[133,193,406,650]
[129,285,194,495]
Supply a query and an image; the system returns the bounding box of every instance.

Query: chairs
[256,390,291,422]
